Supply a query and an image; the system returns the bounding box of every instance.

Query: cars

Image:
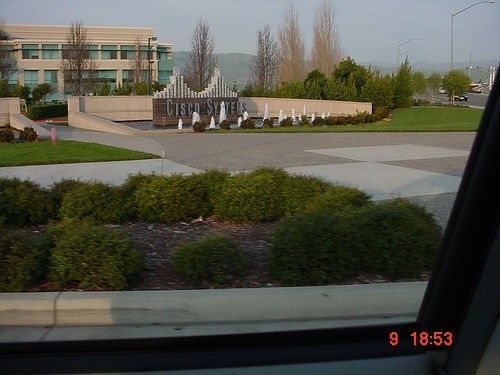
[438,87,447,94]
[448,92,468,102]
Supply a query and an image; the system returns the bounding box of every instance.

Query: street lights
[147,36,158,96]
[397,36,423,75]
[449,1,496,74]
